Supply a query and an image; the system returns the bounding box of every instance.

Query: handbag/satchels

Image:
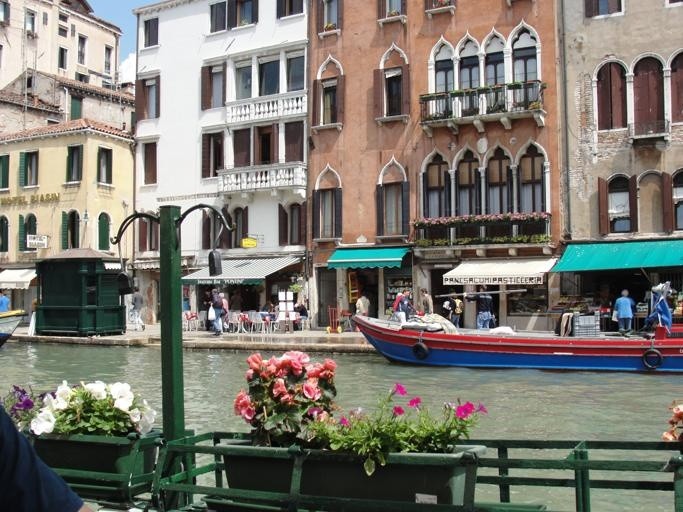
[222,317,230,329]
[611,309,619,323]
[220,308,228,317]
[207,304,216,322]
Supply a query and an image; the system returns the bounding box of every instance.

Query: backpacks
[454,298,465,314]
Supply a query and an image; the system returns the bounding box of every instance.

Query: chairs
[185,309,279,335]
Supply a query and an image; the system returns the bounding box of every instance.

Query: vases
[214,440,488,511]
[26,429,159,501]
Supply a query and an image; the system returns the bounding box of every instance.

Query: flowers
[657,399,683,449]
[304,379,491,477]
[233,346,340,445]
[1,367,154,436]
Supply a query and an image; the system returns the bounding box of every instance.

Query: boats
[350,314,683,373]
[0,309,30,349]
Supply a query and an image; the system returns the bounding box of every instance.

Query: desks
[633,311,683,335]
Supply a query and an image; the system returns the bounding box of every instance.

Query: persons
[390,284,496,329]
[30,293,42,313]
[0,403,97,511]
[200,288,310,338]
[0,288,11,313]
[612,288,636,332]
[352,291,371,332]
[130,286,145,332]
